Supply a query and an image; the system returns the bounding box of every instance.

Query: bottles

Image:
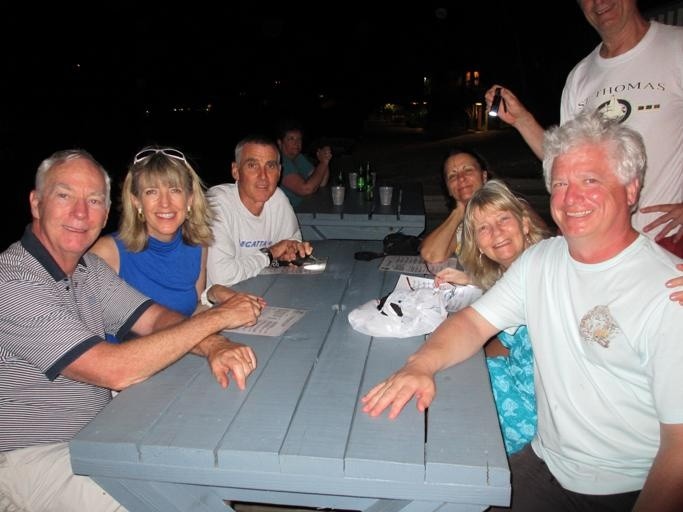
[334,167,346,188]
[357,161,377,199]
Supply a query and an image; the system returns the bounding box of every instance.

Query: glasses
[131,147,187,165]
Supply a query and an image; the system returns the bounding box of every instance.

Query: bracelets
[257,247,276,266]
[199,284,214,306]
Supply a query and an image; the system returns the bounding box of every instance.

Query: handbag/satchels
[355,233,423,261]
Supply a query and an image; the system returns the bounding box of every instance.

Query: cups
[380,186,393,205]
[331,187,344,205]
[349,173,357,190]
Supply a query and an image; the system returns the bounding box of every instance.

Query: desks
[68,239,512,512]
[294,181,426,239]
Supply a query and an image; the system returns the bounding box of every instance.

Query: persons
[433,179,550,455]
[415,144,495,265]
[0,148,261,511]
[83,146,265,342]
[273,124,331,211]
[355,103,681,512]
[205,133,314,291]
[479,1,682,258]
